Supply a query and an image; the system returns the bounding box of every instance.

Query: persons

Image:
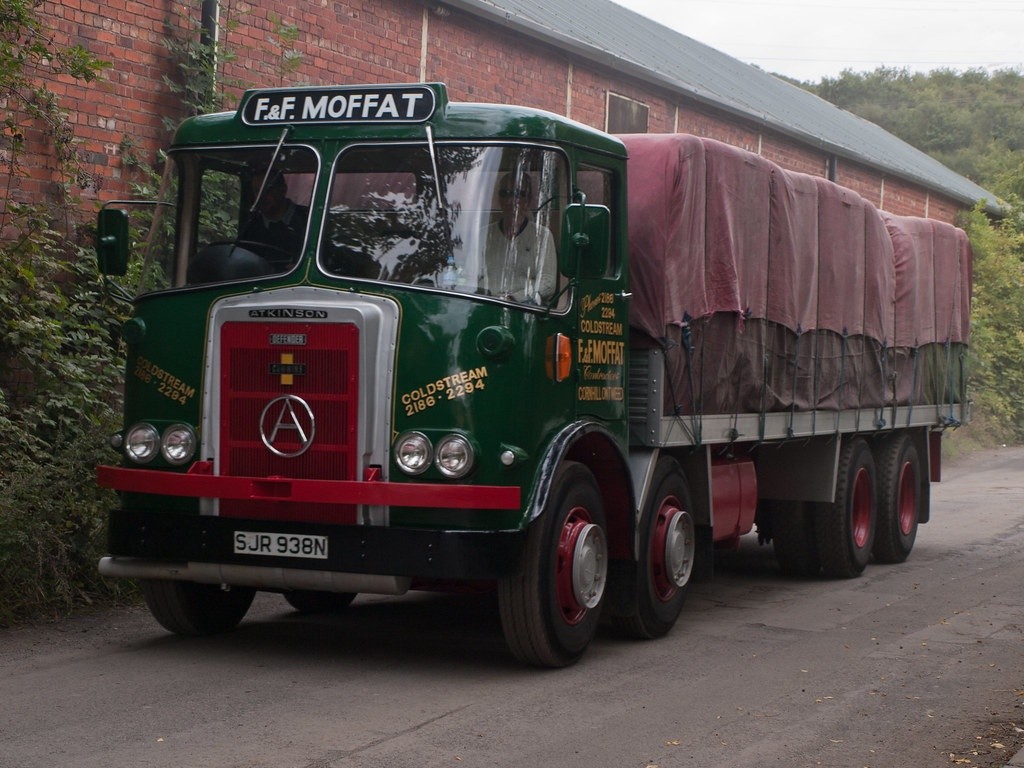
[236,167,338,272]
[463,170,559,306]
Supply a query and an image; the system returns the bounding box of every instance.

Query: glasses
[497,189,530,199]
[251,184,280,197]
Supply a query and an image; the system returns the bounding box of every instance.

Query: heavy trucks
[89,81,976,670]
